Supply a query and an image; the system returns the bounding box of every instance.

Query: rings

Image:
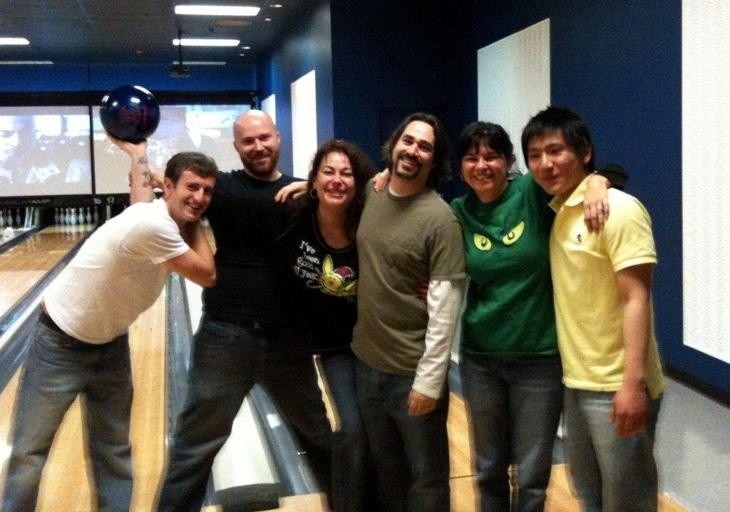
[597,207,604,214]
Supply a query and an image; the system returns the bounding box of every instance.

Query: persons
[273,112,468,511]
[371,121,632,512]
[98,109,342,511]
[2,149,220,510]
[281,137,366,510]
[522,104,666,512]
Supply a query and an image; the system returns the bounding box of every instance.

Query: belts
[212,312,265,331]
[37,310,73,337]
